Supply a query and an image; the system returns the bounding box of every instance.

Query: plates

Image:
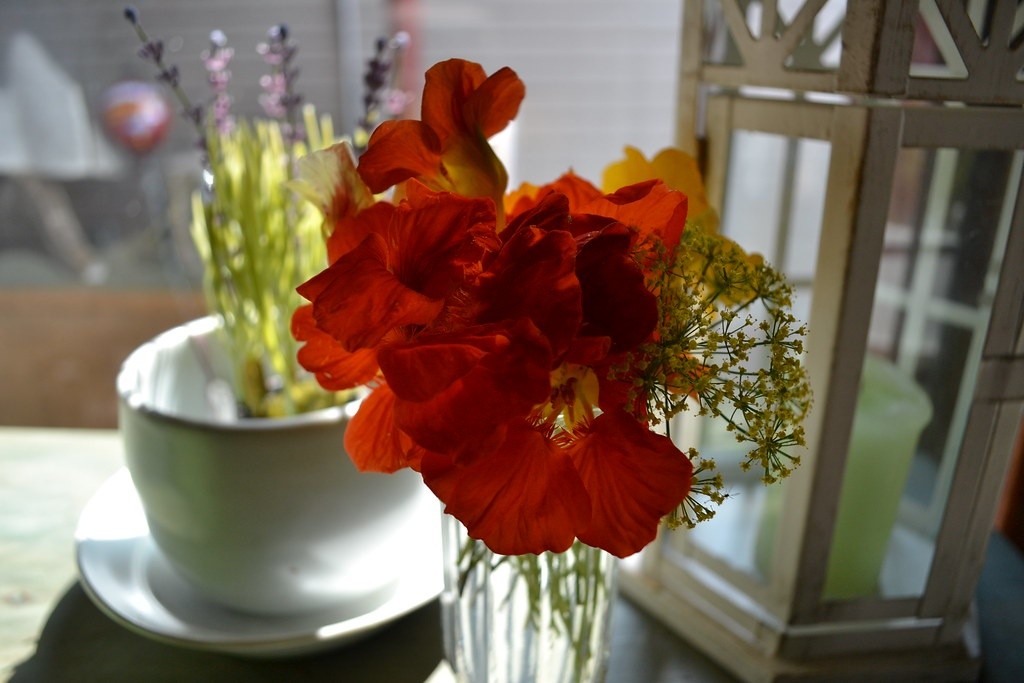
[76,468,446,653]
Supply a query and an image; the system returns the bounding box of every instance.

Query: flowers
[276,57,819,672]
[123,8,427,417]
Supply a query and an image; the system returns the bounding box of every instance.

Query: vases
[438,503,614,683]
[70,307,450,656]
